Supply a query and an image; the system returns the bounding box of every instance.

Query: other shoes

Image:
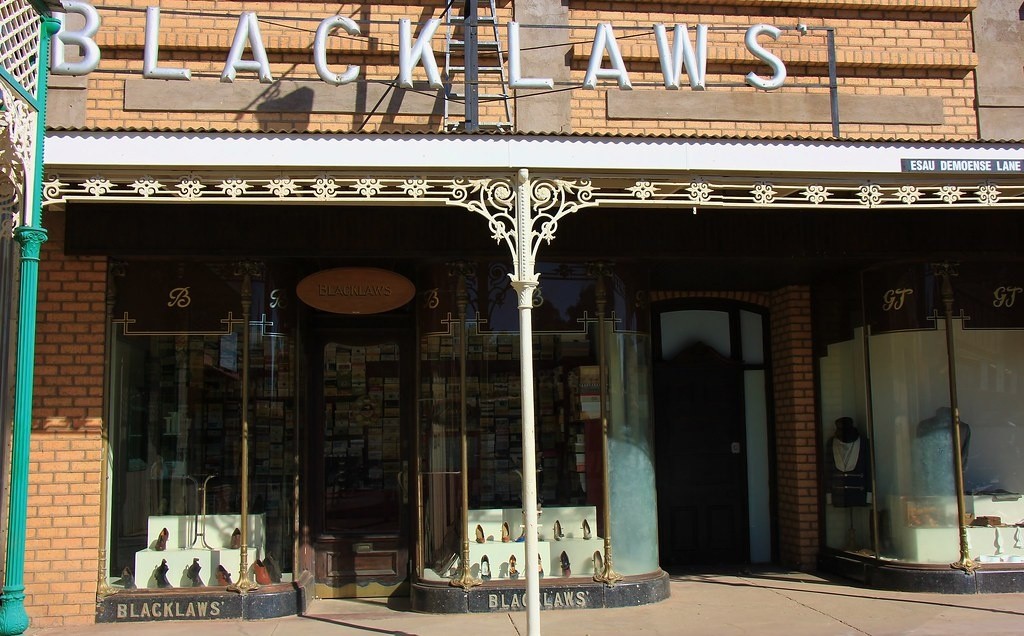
[215,564,232,586]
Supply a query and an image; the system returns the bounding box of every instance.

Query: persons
[824,417,873,508]
[917,405,971,494]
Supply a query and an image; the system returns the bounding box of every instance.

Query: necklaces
[836,439,855,470]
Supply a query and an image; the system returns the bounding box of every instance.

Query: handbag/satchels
[965,513,974,525]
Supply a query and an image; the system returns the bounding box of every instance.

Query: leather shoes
[262,558,280,583]
[120,566,137,589]
[252,558,271,585]
[267,554,282,578]
[230,527,241,549]
[186,558,206,588]
[153,559,173,589]
[155,527,169,551]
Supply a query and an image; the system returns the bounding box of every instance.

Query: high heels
[433,550,461,578]
[592,550,604,573]
[475,524,494,544]
[538,553,545,579]
[509,554,520,579]
[560,550,572,577]
[553,519,563,541]
[582,518,593,540]
[502,521,510,543]
[481,554,492,580]
[514,533,525,543]
